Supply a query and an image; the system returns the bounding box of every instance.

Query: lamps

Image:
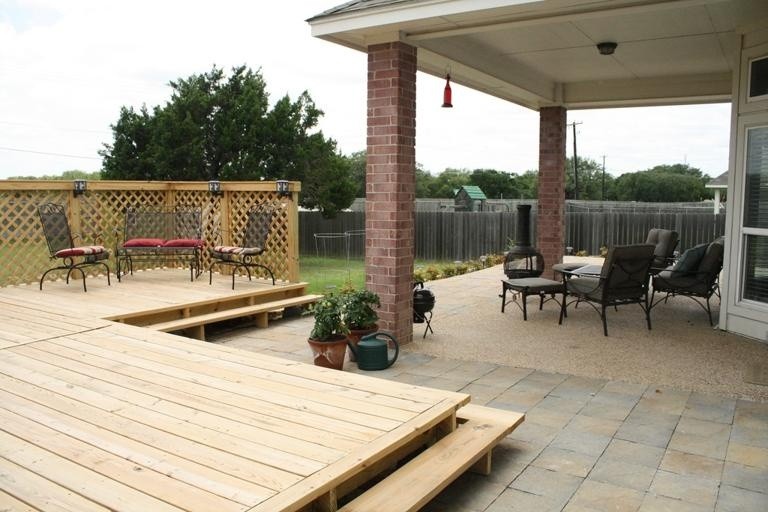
[72,179,88,198]
[208,180,225,198]
[439,63,455,109]
[274,180,293,197]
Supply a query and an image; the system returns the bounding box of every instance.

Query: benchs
[112,202,205,284]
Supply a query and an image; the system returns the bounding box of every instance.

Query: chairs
[552,225,725,338]
[35,202,113,292]
[205,202,278,291]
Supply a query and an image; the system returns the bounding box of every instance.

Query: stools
[499,276,567,323]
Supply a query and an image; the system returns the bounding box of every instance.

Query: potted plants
[307,293,350,372]
[344,289,383,363]
